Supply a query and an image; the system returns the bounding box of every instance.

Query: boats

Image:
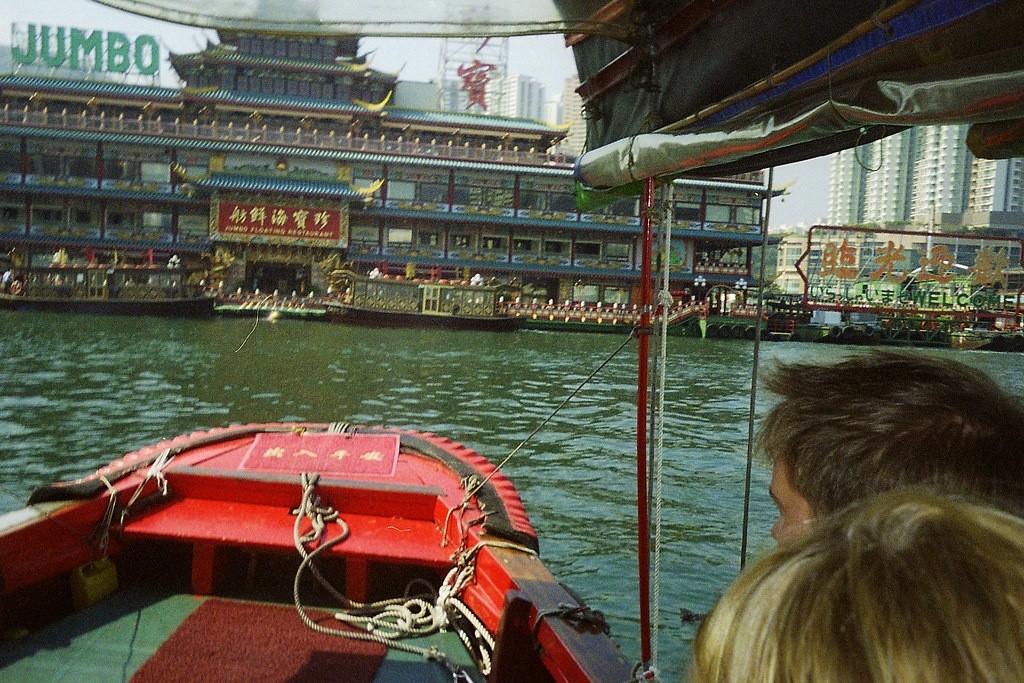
[319,250,529,333]
[0,248,237,318]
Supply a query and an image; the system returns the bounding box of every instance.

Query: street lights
[733,278,748,314]
[693,275,706,305]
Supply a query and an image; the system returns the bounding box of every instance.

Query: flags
[380,261,470,283]
[46,249,158,268]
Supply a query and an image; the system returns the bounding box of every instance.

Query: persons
[691,345,1024,683]
